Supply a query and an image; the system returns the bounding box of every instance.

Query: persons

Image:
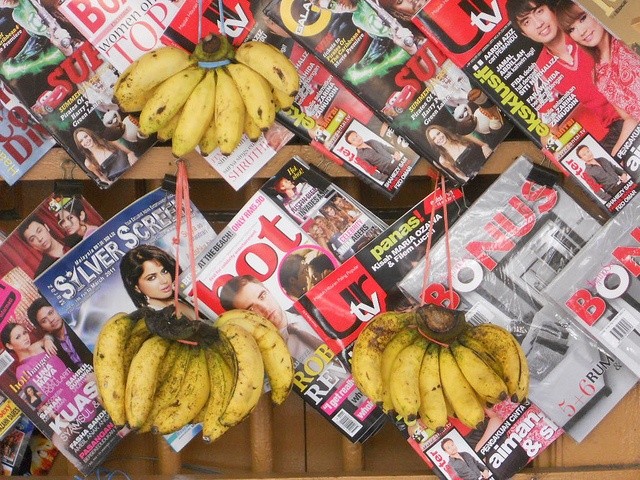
[275,177,322,219]
[554,0,640,157]
[314,216,334,240]
[280,254,308,298]
[0,323,97,468]
[310,1,418,56]
[332,195,360,221]
[308,222,332,254]
[322,203,349,230]
[309,254,334,286]
[120,244,205,320]
[40,1,86,55]
[345,131,400,181]
[499,0,640,184]
[575,145,628,202]
[425,125,493,182]
[441,439,492,480]
[0,0,54,37]
[17,216,82,279]
[25,386,60,423]
[73,128,137,185]
[27,296,93,373]
[21,426,58,476]
[218,276,350,382]
[57,201,98,240]
[248,0,398,144]
[378,1,473,113]
[385,290,529,479]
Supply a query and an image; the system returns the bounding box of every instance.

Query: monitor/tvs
[574,1,639,57]
[409,0,640,217]
[0,417,35,476]
[294,187,566,480]
[543,193,639,382]
[172,157,391,445]
[0,191,134,476]
[293,158,390,443]
[0,75,58,186]
[400,156,640,443]
[0,392,23,441]
[34,184,217,454]
[291,157,391,438]
[261,0,516,183]
[57,0,295,193]
[0,1,160,191]
[162,0,421,196]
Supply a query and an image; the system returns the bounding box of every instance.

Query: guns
[93,304,294,443]
[352,303,529,433]
[111,32,300,158]
